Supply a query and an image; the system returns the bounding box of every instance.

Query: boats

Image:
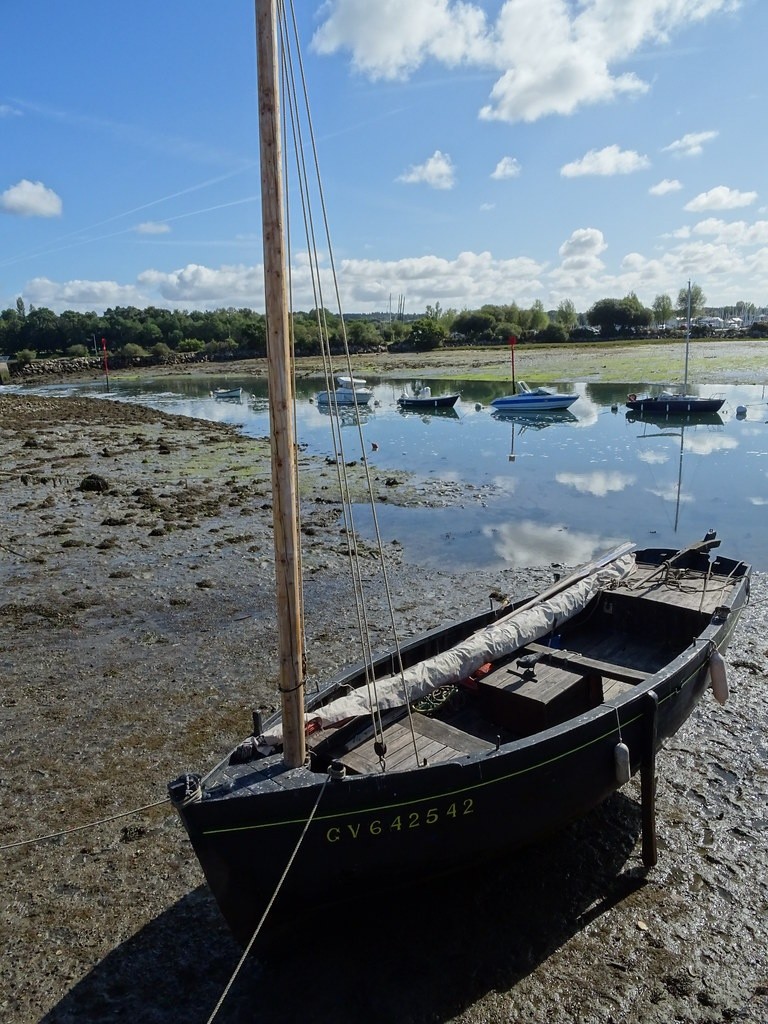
[214,387,242,397]
[316,388,373,405]
[397,406,460,424]
[490,381,580,411]
[337,377,367,388]
[396,387,463,408]
[317,404,376,427]
[490,410,579,436]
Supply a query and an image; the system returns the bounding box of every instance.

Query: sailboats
[627,414,725,534]
[168,0,752,952]
[624,279,727,414]
